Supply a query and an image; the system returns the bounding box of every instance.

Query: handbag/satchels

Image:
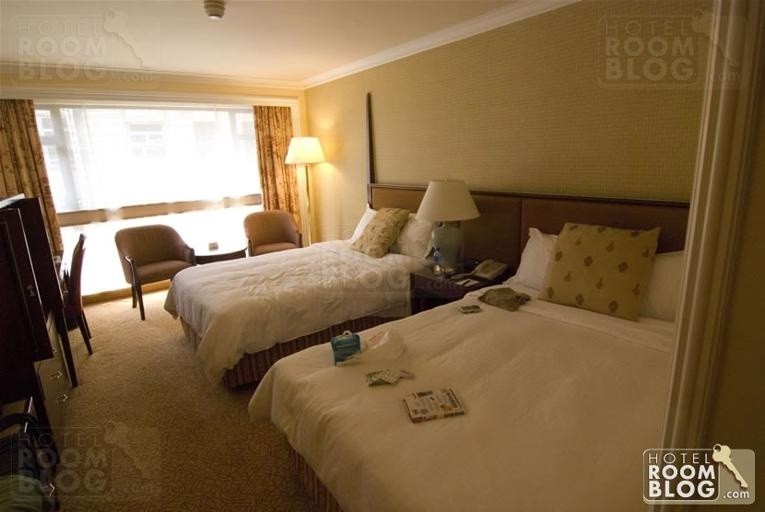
[331,330,361,367]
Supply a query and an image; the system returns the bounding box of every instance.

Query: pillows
[515,227,684,324]
[345,202,441,260]
[350,206,410,257]
[538,222,663,323]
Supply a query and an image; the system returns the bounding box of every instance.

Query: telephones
[468,259,507,281]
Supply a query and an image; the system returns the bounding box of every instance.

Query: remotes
[459,306,481,314]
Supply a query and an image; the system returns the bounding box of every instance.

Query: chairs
[113,224,196,319]
[59,235,92,387]
[243,209,304,256]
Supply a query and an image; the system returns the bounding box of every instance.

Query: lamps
[414,178,481,262]
[203,0,228,20]
[284,137,325,247]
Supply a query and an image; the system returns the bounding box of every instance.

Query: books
[403,387,465,424]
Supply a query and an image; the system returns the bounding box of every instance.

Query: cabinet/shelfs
[0,192,65,406]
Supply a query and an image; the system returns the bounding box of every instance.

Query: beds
[164,183,522,390]
[188,235,247,266]
[247,192,690,510]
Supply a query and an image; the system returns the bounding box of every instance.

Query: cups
[53,256,60,264]
[208,242,219,252]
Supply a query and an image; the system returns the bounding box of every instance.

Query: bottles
[433,247,445,281]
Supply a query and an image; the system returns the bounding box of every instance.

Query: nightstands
[410,264,485,315]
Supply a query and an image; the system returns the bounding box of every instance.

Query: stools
[0,393,56,479]
